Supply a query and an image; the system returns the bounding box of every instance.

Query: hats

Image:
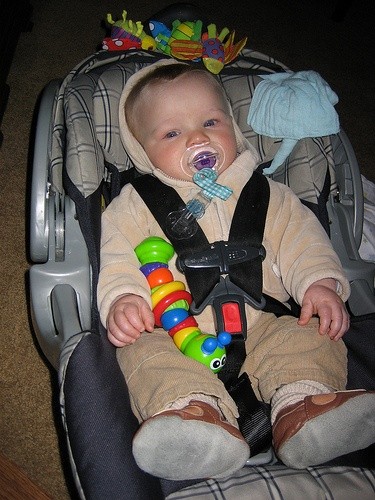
[246,70,341,175]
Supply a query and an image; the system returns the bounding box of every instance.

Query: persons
[96,59,375,482]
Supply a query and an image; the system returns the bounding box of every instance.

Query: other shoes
[132,400,251,481]
[269,387,374,469]
